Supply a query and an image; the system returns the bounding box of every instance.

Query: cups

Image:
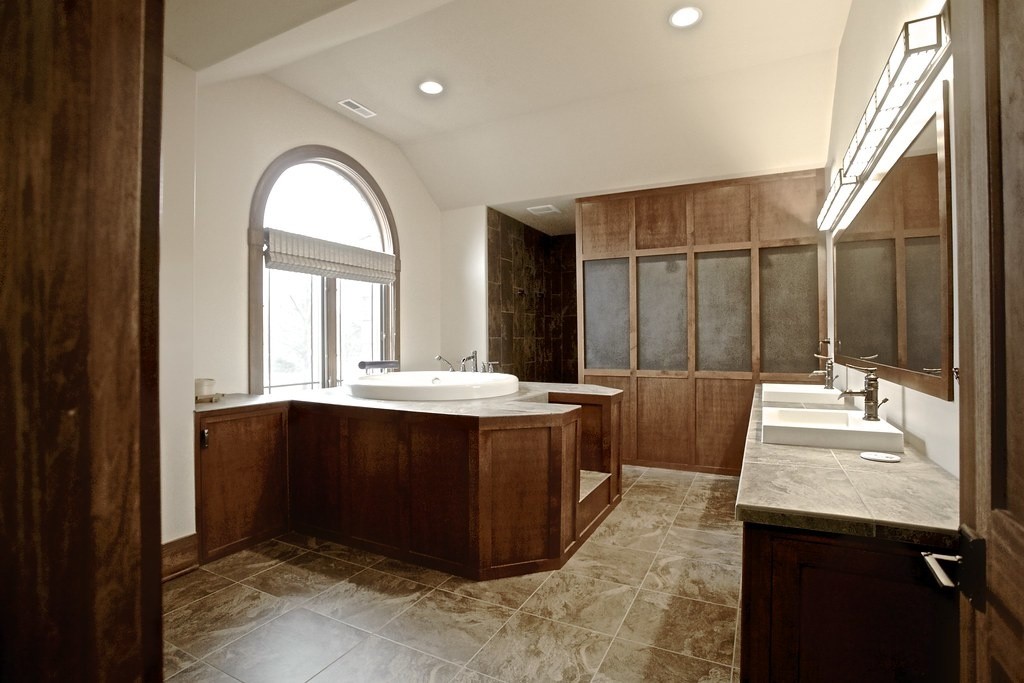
[195,379,215,395]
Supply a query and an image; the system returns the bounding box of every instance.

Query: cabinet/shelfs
[194,402,292,567]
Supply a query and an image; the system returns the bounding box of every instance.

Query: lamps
[816,0,951,231]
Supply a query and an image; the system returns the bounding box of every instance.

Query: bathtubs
[343,370,519,401]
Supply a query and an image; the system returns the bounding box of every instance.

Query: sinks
[761,406,904,453]
[762,383,844,405]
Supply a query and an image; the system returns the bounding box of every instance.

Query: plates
[860,450,901,462]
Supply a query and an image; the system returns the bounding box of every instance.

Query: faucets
[461,349,478,372]
[434,355,454,372]
[837,363,879,421]
[809,353,834,389]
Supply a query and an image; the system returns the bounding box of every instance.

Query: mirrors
[832,81,955,401]
